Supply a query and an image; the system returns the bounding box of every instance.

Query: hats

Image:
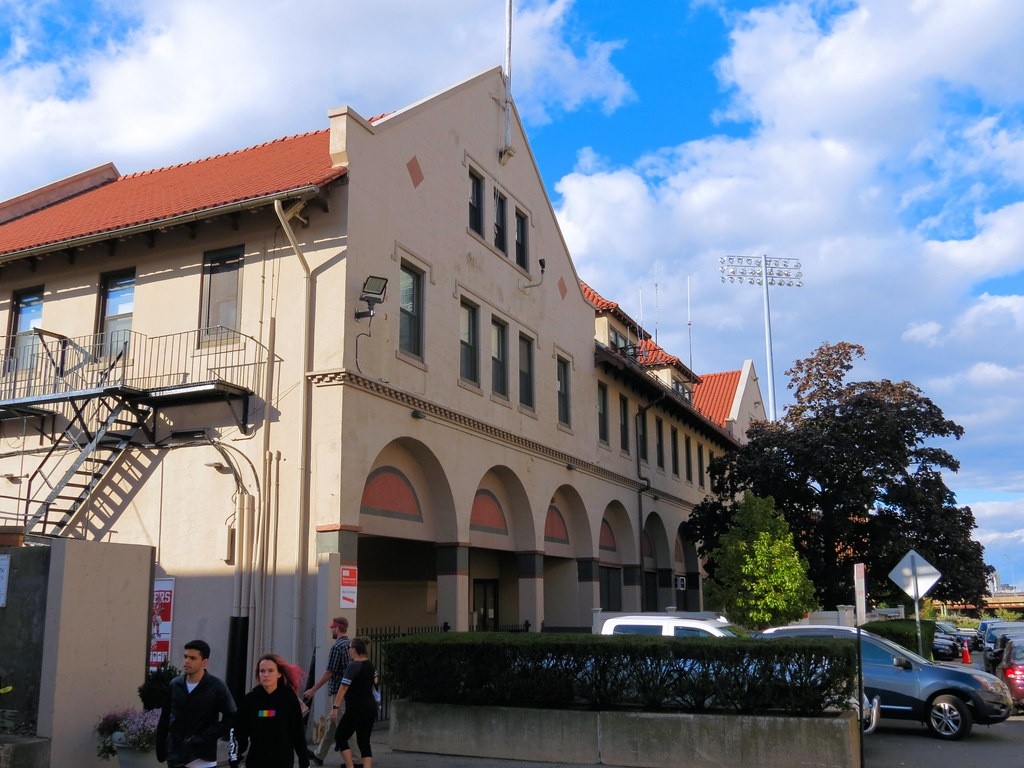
[328,620,348,628]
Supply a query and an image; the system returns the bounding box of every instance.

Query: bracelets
[332,706,340,710]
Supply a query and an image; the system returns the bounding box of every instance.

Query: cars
[931,617,1024,717]
[599,614,1012,740]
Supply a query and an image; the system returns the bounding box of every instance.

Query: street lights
[718,252,804,421]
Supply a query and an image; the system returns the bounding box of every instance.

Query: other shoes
[340,763,364,768]
[307,749,324,766]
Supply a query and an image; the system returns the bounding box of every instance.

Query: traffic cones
[962,638,973,664]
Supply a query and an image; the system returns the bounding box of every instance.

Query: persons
[302,617,364,768]
[155,640,237,768]
[228,654,310,768]
[326,636,379,768]
